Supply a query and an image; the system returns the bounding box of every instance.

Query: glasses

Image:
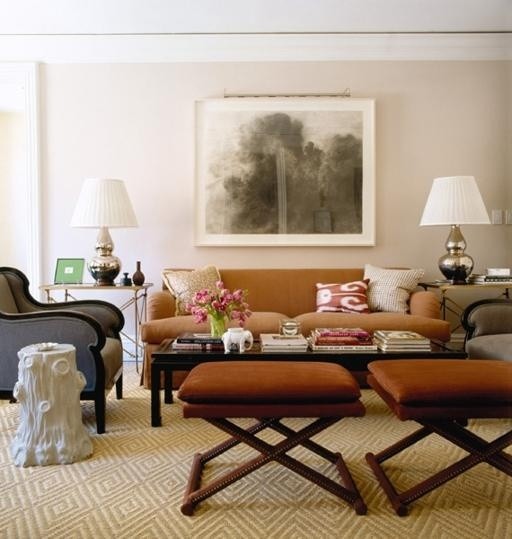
[70,179,138,286]
[419,175,492,285]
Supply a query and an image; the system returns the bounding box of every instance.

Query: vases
[185,280,252,328]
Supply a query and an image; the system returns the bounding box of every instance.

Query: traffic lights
[363,263,425,316]
[312,279,372,314]
[160,264,222,317]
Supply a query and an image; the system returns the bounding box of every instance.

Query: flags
[210,317,225,336]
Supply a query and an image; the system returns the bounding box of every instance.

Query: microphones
[365,359,512,517]
[177,361,369,516]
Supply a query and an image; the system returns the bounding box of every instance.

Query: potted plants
[192,95,376,247]
[54,257,85,284]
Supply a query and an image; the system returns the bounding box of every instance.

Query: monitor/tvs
[172,333,236,351]
[306,327,378,352]
[258,333,308,352]
[468,273,512,285]
[373,330,432,352]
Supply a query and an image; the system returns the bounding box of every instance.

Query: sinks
[460,298,512,360]
[0,267,125,434]
[139,268,452,389]
